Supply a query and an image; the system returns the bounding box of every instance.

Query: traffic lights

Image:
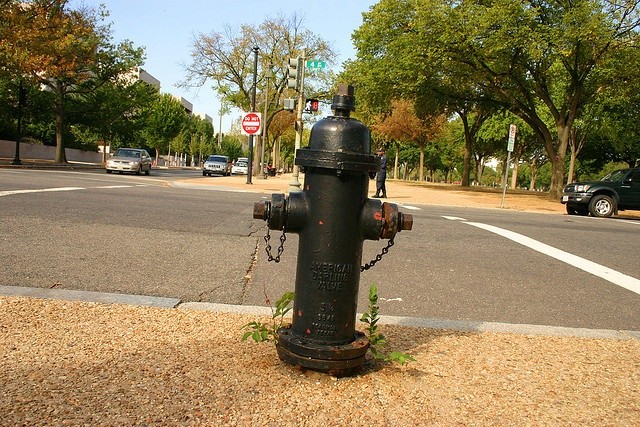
[287,56,299,90]
[305,99,318,111]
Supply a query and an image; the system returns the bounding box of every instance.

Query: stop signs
[241,112,262,135]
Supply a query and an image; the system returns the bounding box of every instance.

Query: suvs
[562,167,640,218]
[203,155,230,176]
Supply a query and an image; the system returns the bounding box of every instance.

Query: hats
[377,148,385,152]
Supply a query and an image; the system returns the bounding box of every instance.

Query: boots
[379,191,387,198]
[371,191,380,198]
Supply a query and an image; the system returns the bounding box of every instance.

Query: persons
[371,148,386,198]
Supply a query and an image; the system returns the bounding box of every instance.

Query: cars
[106,148,152,176]
[231,162,248,175]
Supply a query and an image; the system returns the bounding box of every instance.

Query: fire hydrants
[253,84,413,376]
